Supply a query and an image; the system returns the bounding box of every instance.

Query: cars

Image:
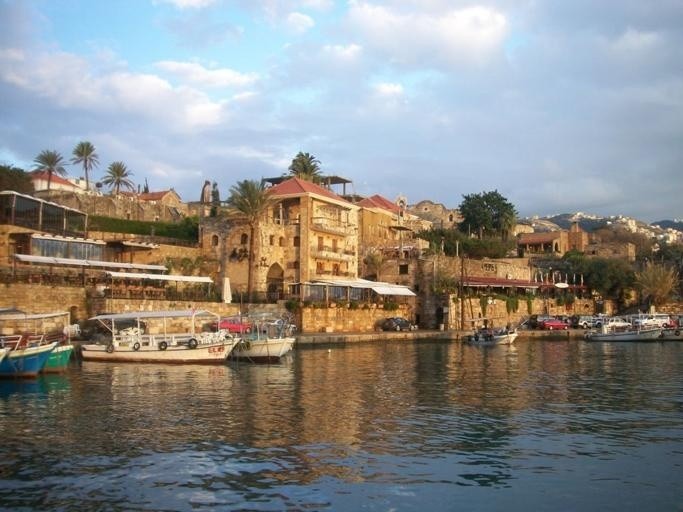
[382,317,415,331]
[93,312,147,337]
[257,319,297,335]
[212,317,253,333]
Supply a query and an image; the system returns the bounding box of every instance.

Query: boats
[0,305,77,379]
[643,314,682,340]
[459,314,519,345]
[81,308,238,366]
[588,318,662,341]
[215,310,298,360]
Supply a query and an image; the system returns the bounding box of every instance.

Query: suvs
[528,313,682,330]
